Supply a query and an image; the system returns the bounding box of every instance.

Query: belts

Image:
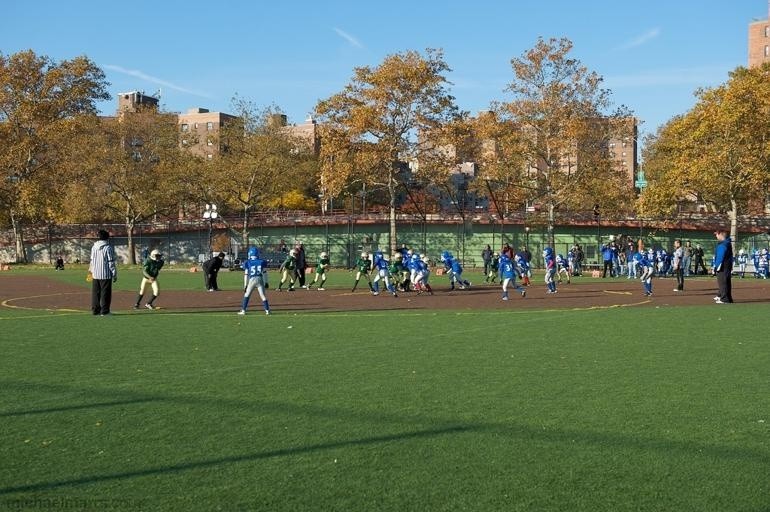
[145,276,149,280]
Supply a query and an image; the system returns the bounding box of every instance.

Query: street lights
[525,226,530,250]
[320,193,334,215]
[201,199,220,260]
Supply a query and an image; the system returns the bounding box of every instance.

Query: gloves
[264,283,269,289]
[113,276,117,283]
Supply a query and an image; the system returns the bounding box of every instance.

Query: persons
[55,255,64,270]
[291,241,307,289]
[237,246,272,315]
[759,247,770,280]
[275,248,298,291]
[279,239,288,252]
[306,252,330,291]
[712,230,734,304]
[202,252,225,293]
[88,230,116,317]
[133,249,165,310]
[751,250,761,278]
[710,230,730,300]
[234,258,240,265]
[143,247,150,265]
[736,248,748,280]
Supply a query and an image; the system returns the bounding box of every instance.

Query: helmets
[394,252,402,262]
[289,249,299,260]
[247,247,260,260]
[412,254,420,262]
[149,249,163,262]
[440,250,449,259]
[633,253,643,261]
[320,252,329,260]
[423,256,430,265]
[543,247,553,256]
[360,252,369,261]
[374,251,383,260]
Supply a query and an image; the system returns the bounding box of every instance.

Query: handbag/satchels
[85,272,93,282]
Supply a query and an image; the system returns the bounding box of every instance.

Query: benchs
[230,251,288,270]
[579,263,628,277]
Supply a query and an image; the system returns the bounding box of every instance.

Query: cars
[197,247,291,272]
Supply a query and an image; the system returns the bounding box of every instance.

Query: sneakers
[274,285,326,292]
[350,278,475,297]
[144,302,154,310]
[713,295,724,304]
[265,309,270,315]
[133,303,140,310]
[207,288,214,291]
[485,278,574,302]
[237,309,246,315]
[94,311,114,317]
[673,288,683,292]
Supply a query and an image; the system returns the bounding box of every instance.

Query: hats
[98,229,109,240]
